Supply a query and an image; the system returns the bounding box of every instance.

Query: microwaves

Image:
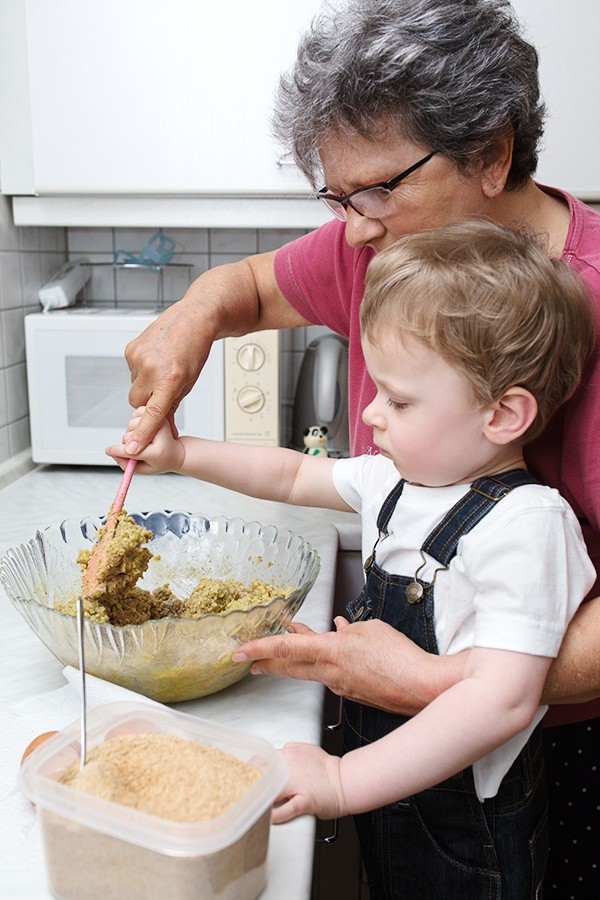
[23,305,286,471]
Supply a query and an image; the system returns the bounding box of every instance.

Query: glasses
[316,149,440,222]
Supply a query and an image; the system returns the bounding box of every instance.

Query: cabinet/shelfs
[0,0,600,230]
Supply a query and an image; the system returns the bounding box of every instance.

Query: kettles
[291,333,351,458]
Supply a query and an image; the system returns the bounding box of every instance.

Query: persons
[126,0,600,900]
[103,217,600,900]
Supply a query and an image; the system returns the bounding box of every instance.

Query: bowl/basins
[0,510,323,702]
[17,700,290,899]
